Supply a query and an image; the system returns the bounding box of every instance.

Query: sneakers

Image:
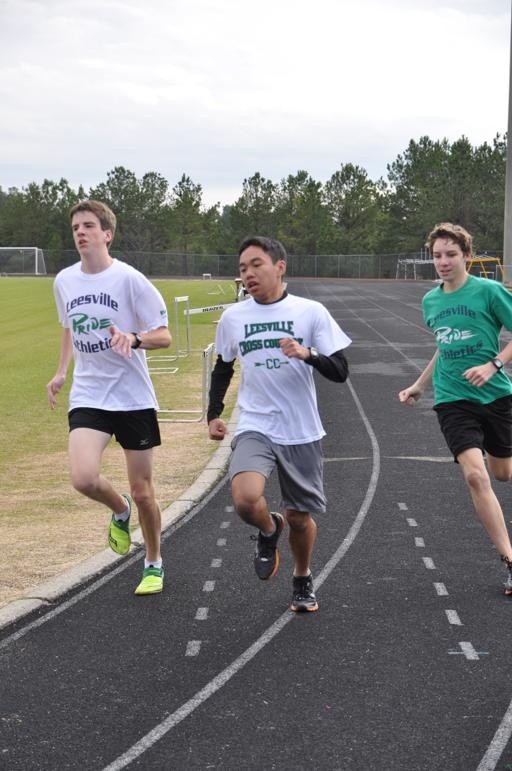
[134,565,166,595]
[107,493,132,557]
[252,512,285,581]
[290,569,319,614]
[501,554,512,597]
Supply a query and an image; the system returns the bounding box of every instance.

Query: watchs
[489,357,504,373]
[130,331,142,350]
[304,346,318,365]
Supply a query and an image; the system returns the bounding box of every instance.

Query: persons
[398,222,512,597]
[206,234,355,613]
[45,201,171,596]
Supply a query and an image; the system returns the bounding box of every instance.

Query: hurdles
[137,288,245,423]
